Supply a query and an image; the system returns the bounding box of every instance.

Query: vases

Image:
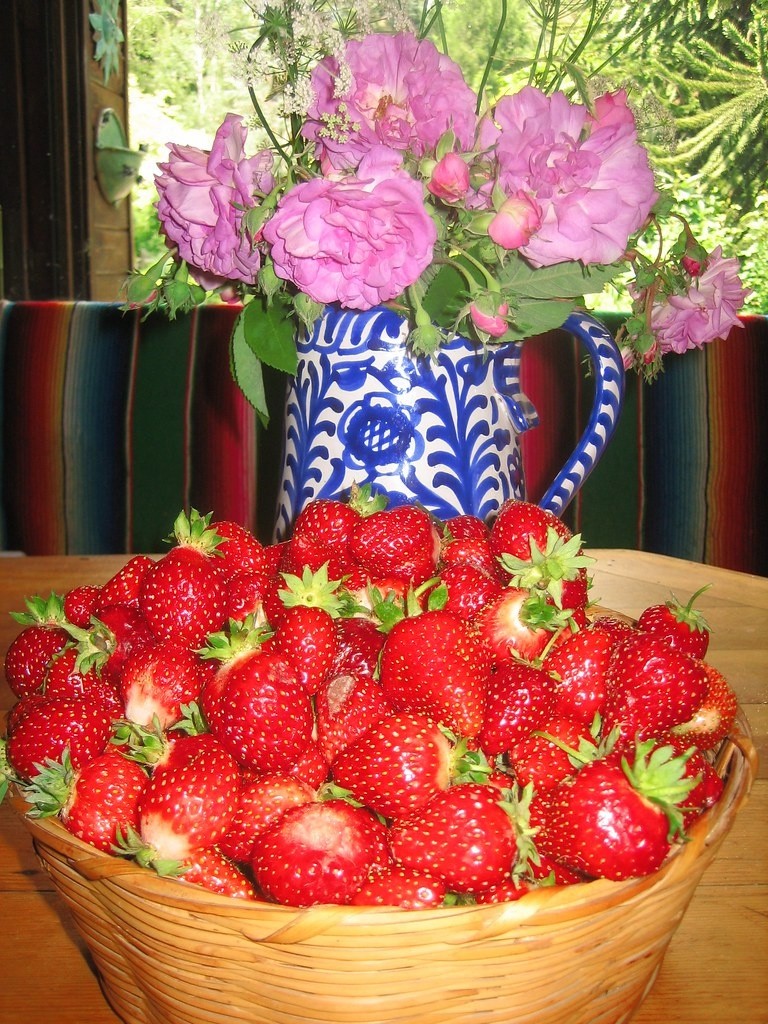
[270,296,625,551]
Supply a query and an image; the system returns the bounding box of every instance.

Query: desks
[1,545,768,1021]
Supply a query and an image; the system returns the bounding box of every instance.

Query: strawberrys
[0,477,738,908]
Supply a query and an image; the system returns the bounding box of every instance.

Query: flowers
[157,29,749,374]
